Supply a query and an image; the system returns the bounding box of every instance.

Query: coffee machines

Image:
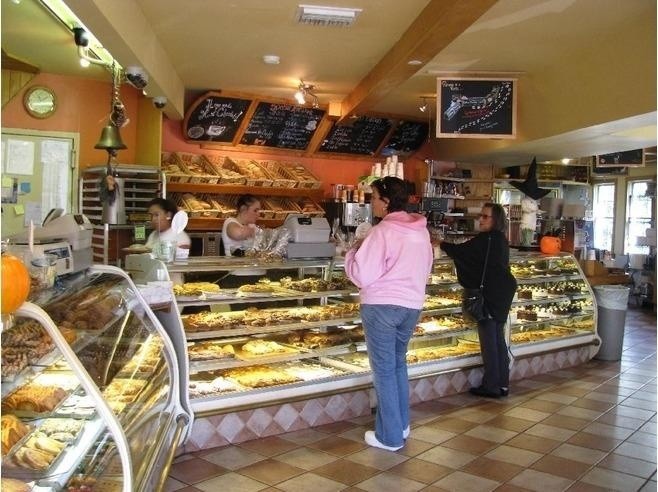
[326,202,372,229]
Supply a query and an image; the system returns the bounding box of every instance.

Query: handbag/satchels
[461,234,491,323]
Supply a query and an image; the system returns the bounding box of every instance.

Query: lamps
[294,77,322,111]
[418,102,428,112]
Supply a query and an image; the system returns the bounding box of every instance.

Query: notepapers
[14,205,25,215]
[2,178,12,188]
[135,226,148,241]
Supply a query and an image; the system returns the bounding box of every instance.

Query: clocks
[23,84,58,120]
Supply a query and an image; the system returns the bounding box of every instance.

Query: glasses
[478,213,495,220]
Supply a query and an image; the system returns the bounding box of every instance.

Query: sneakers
[469,386,501,397]
[364,430,404,451]
[499,387,511,396]
[399,426,412,437]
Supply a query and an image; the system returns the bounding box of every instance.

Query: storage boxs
[290,195,326,220]
[279,159,320,190]
[225,156,273,188]
[263,194,300,220]
[179,192,222,218]
[174,151,220,184]
[206,192,241,219]
[227,194,275,219]
[161,151,192,182]
[166,191,190,216]
[252,158,298,187]
[202,153,247,185]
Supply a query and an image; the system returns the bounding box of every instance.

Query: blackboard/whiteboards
[590,156,628,178]
[374,117,434,162]
[436,77,518,140]
[234,96,326,155]
[596,148,645,168]
[313,114,393,159]
[187,91,257,147]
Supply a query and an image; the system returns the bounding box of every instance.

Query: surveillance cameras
[153,96,167,110]
[125,66,148,89]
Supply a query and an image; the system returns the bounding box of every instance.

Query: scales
[119,248,152,271]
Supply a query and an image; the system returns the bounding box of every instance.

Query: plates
[237,284,272,297]
[104,378,148,405]
[175,294,200,302]
[6,435,67,475]
[146,344,164,357]
[119,356,160,378]
[35,418,84,446]
[1,381,70,419]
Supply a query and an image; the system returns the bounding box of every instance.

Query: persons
[431,202,518,398]
[144,197,192,315]
[343,176,434,453]
[221,193,273,312]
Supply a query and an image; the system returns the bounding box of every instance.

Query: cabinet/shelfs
[426,160,493,236]
[125,246,603,454]
[2,264,192,487]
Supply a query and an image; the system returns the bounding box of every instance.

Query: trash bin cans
[593,285,631,360]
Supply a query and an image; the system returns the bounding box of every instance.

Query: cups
[371,155,404,180]
